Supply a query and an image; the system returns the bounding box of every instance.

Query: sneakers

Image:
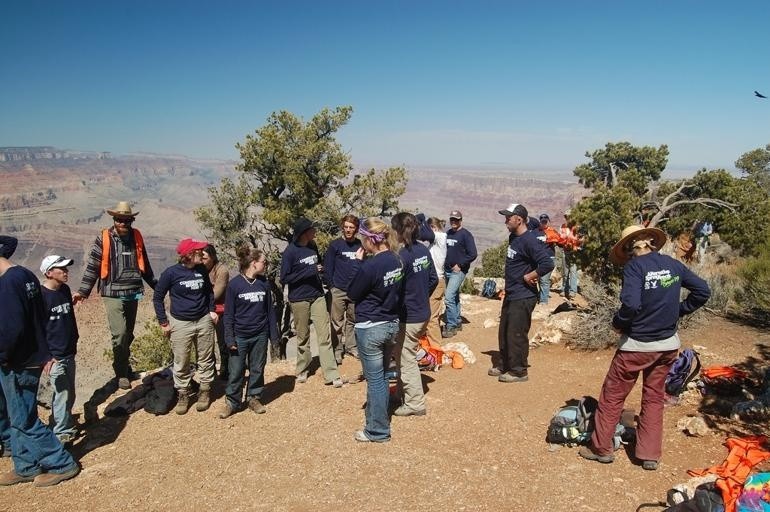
[345,346,360,359]
[247,398,266,414]
[332,379,343,388]
[336,359,342,364]
[33,465,80,487]
[297,370,309,383]
[643,460,657,470]
[219,404,233,418]
[488,367,502,376]
[442,328,457,338]
[176,395,190,415]
[498,371,528,383]
[115,376,130,390]
[393,404,426,416]
[0,469,36,486]
[354,431,369,442]
[195,390,212,410]
[578,448,615,463]
[456,323,462,331]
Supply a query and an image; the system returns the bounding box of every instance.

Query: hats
[498,204,528,220]
[40,255,74,275]
[540,214,550,223]
[106,201,139,219]
[610,225,667,265]
[175,237,208,257]
[0,236,18,259]
[293,218,322,243]
[449,210,462,219]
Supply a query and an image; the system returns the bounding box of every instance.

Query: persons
[219,248,279,419]
[578,223,710,470]
[39,255,80,433]
[488,203,554,382]
[0,236,81,488]
[441,210,477,338]
[417,217,447,350]
[153,239,218,414]
[560,209,579,299]
[279,217,344,388]
[392,213,439,417]
[202,244,229,378]
[322,215,364,365]
[347,217,405,441]
[70,201,158,389]
[692,219,713,266]
[532,214,560,305]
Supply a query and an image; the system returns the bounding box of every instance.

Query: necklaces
[240,273,257,286]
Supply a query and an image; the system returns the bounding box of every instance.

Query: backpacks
[480,279,496,299]
[664,348,702,397]
[545,396,635,451]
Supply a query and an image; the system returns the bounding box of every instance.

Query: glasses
[46,256,66,271]
[115,217,132,223]
